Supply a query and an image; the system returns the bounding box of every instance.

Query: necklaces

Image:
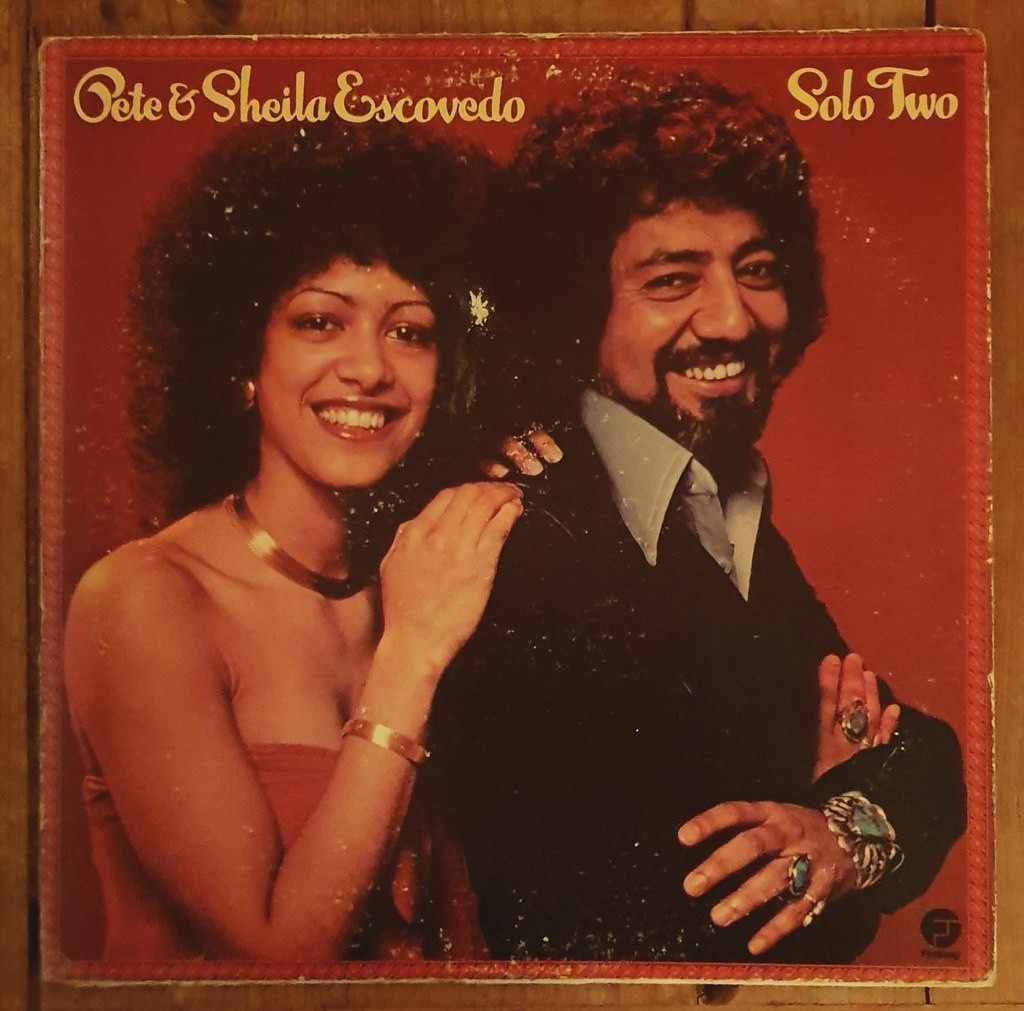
[225,491,374,601]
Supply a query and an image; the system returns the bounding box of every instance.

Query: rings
[775,853,813,903]
[837,697,873,747]
[802,894,828,928]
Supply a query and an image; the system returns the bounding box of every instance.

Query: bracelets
[824,791,897,889]
[341,719,428,767]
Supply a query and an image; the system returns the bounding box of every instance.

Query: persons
[65,123,563,963]
[396,78,965,963]
